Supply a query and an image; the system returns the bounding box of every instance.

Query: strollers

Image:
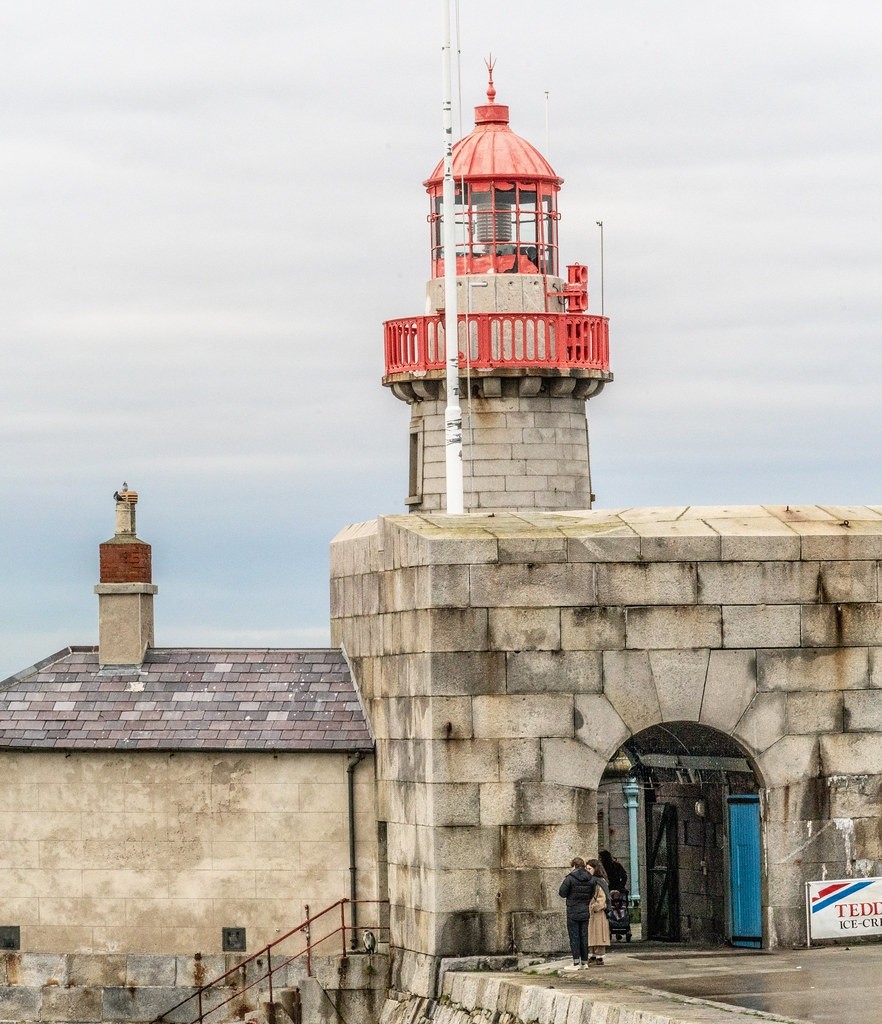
[608,889,632,942]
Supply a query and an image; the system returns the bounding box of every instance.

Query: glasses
[586,867,594,871]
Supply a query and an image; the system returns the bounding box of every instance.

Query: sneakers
[563,964,580,970]
[580,965,589,970]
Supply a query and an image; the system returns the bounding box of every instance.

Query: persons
[584,859,610,965]
[559,857,596,971]
[608,890,626,921]
[598,850,628,941]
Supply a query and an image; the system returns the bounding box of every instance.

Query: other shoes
[589,957,597,964]
[593,958,604,967]
[616,935,622,941]
